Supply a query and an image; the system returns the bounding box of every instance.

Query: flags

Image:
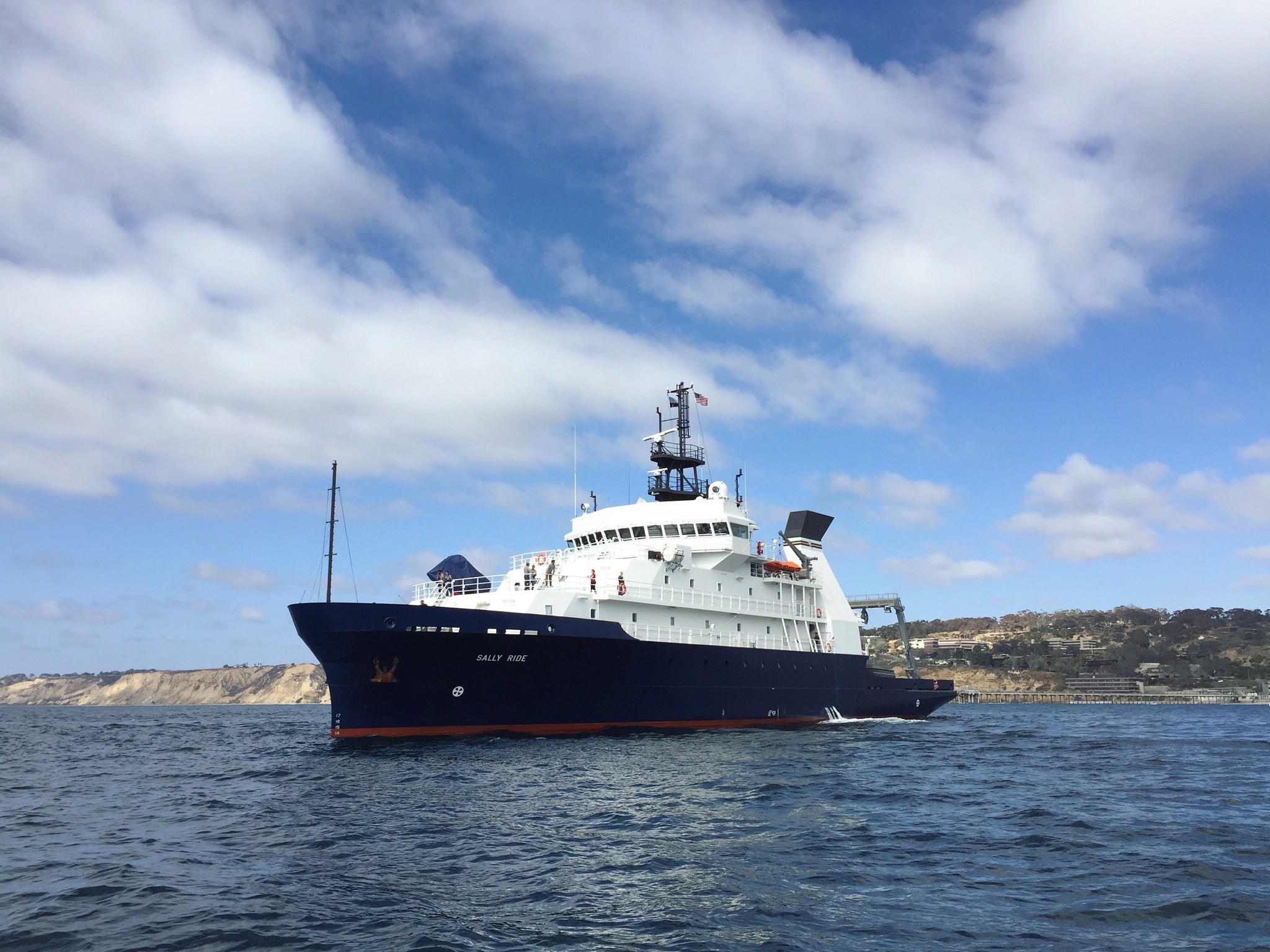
[694,392,708,406]
[669,396,678,407]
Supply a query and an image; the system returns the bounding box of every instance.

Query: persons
[524,563,531,590]
[437,570,444,596]
[420,600,427,606]
[587,569,597,595]
[814,632,820,652]
[445,571,453,596]
[618,572,625,585]
[545,560,555,587]
[530,565,537,590]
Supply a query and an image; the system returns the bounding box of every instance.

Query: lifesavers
[619,582,626,595]
[758,542,761,553]
[816,608,821,618]
[537,554,546,565]
[826,643,831,652]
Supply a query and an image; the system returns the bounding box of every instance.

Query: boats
[764,559,801,573]
[286,380,959,739]
